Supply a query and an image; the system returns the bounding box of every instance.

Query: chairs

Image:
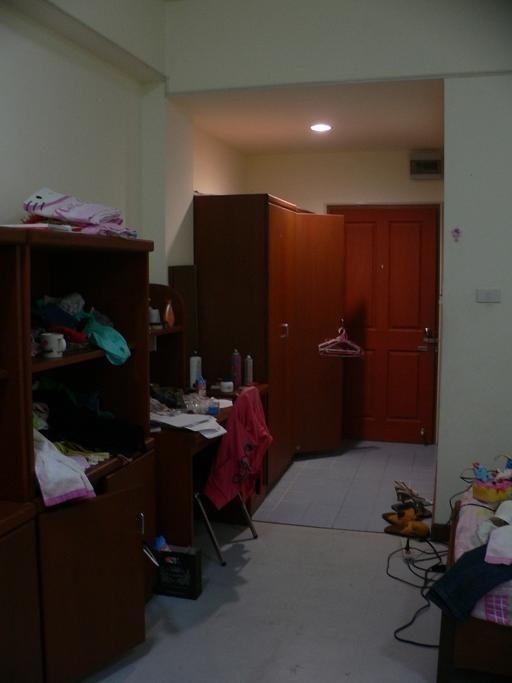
[193,386,258,563]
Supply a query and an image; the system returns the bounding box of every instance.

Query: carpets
[252,438,437,534]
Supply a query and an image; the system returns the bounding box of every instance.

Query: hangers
[319,326,364,358]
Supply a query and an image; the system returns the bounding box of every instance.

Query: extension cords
[400,537,415,562]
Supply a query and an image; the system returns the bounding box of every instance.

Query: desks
[149,283,270,546]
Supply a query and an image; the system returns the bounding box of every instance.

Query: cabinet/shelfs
[295,203,345,453]
[0,226,34,503]
[0,519,46,683]
[35,439,157,680]
[23,225,153,501]
[193,193,294,496]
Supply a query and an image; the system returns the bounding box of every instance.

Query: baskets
[472,453,512,503]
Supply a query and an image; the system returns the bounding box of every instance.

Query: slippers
[383,507,417,526]
[383,521,431,538]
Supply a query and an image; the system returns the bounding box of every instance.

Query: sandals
[391,480,432,519]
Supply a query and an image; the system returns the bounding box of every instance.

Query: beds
[437,500,511,682]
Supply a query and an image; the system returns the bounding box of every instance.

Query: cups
[42,333,66,358]
